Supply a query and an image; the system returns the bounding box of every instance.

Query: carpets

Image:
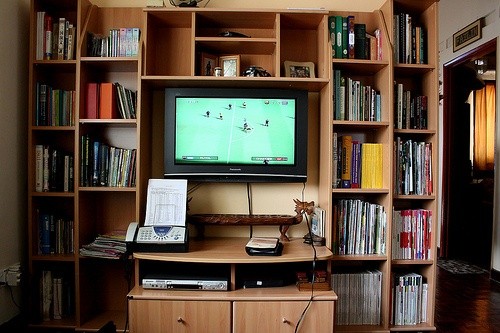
[436,259,489,275]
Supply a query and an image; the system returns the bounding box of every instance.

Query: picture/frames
[219,56,240,77]
[201,52,218,76]
[283,60,315,79]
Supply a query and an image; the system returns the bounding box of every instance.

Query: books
[31,9,137,324]
[328,9,436,328]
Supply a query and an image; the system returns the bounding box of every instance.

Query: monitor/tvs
[165,88,307,183]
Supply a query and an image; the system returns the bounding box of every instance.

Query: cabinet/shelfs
[28,0,439,333]
